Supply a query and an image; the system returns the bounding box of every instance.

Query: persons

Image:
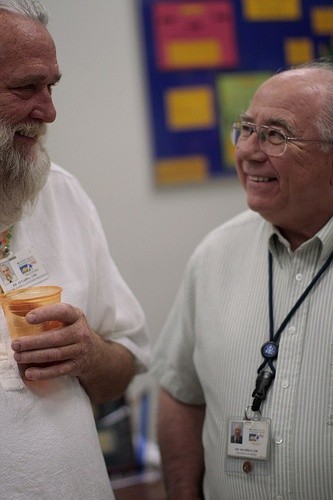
[1,0,158,500]
[230,423,242,444]
[153,62,332,499]
[1,265,17,285]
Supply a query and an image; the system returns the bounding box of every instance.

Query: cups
[0,286,64,384]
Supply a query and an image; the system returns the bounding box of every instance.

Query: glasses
[231,121,333,158]
